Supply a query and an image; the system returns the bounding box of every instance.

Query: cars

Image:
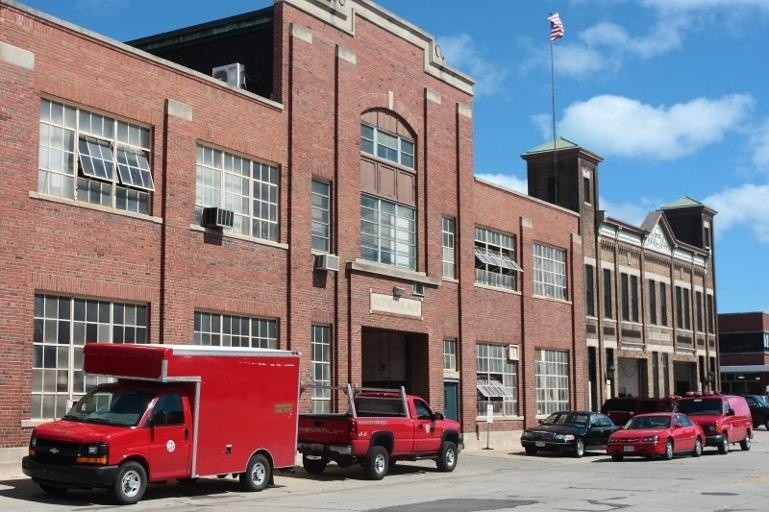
[521,391,769,460]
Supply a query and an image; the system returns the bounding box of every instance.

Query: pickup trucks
[297,384,464,479]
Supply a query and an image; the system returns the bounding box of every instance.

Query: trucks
[23,343,302,505]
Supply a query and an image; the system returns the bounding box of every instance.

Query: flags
[548,12,564,41]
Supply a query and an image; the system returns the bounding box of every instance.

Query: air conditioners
[210,62,249,90]
[316,254,340,273]
[201,206,234,229]
